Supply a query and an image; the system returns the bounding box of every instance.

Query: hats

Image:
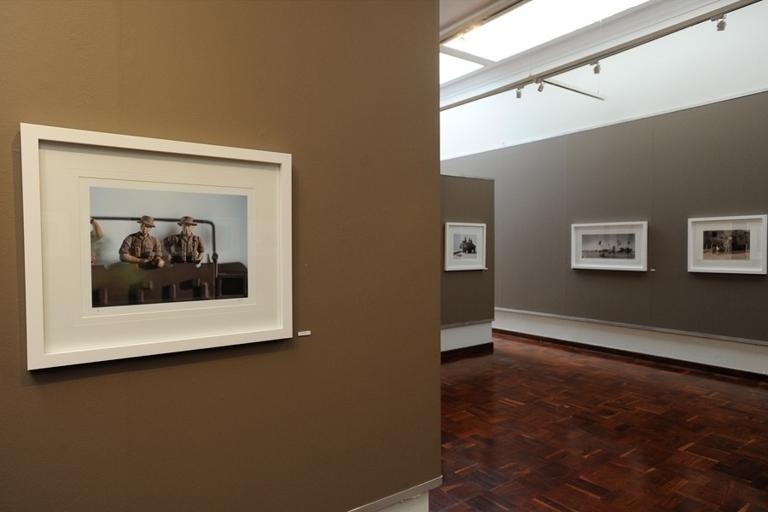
[177,217,197,226]
[137,215,157,229]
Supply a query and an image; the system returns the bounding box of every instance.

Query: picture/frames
[570,214,768,277]
[444,222,489,272]
[20,118,296,373]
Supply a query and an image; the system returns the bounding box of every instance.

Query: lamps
[514,11,729,102]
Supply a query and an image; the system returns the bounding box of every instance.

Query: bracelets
[91,218,94,223]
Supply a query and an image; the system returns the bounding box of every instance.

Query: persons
[90,217,110,264]
[164,216,210,264]
[119,215,165,267]
